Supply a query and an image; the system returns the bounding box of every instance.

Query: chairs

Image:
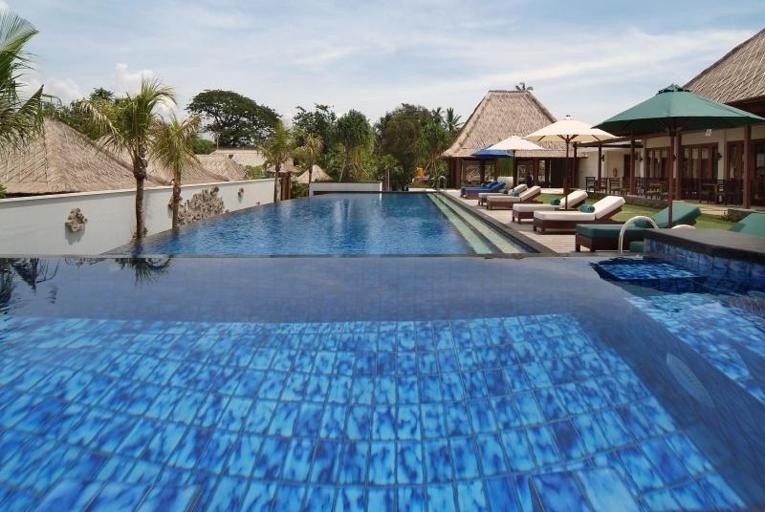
[512,190,587,223]
[729,213,764,235]
[519,175,763,210]
[462,181,499,197]
[574,202,701,253]
[486,186,541,209]
[478,184,528,206]
[464,182,507,198]
[533,195,626,235]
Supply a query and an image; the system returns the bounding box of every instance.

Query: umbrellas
[485,134,545,187]
[589,83,765,229]
[523,112,616,209]
[469,143,514,181]
